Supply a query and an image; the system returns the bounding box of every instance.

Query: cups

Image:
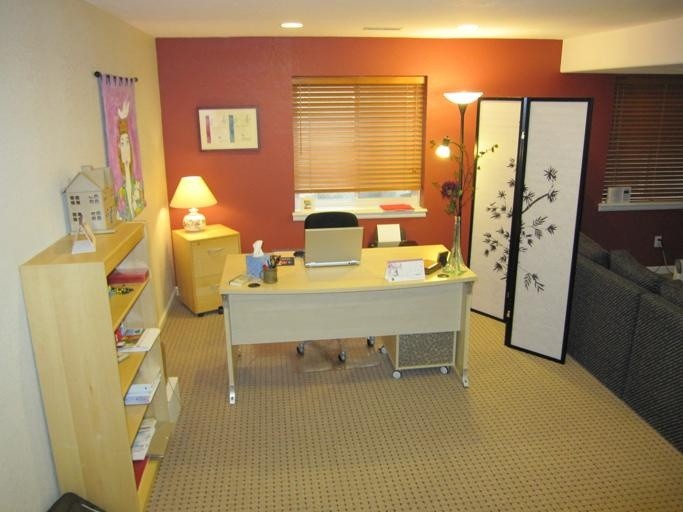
[260,268,277,284]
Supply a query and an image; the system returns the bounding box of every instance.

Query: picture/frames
[195,104,262,154]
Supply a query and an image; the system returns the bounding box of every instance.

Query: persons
[115,94,148,221]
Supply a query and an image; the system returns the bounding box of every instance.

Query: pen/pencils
[267,254,281,268]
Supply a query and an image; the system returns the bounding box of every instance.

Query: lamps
[432,90,485,216]
[169,175,218,233]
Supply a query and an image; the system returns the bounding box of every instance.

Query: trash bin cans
[47,491,105,512]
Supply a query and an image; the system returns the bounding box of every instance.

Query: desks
[219,244,476,405]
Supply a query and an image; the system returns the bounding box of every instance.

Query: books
[124,382,153,404]
[137,417,157,428]
[107,269,148,282]
[130,429,154,461]
[115,327,161,352]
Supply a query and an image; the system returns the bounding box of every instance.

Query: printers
[367,230,417,248]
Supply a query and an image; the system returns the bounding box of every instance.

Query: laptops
[304,227,364,268]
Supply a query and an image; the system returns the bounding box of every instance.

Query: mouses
[294,251,304,257]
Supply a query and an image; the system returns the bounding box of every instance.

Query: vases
[443,216,470,274]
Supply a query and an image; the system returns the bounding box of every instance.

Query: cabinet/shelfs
[19,221,173,512]
[172,223,241,314]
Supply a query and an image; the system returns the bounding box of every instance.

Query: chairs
[296,211,377,361]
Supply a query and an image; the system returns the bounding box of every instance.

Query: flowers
[428,139,499,258]
